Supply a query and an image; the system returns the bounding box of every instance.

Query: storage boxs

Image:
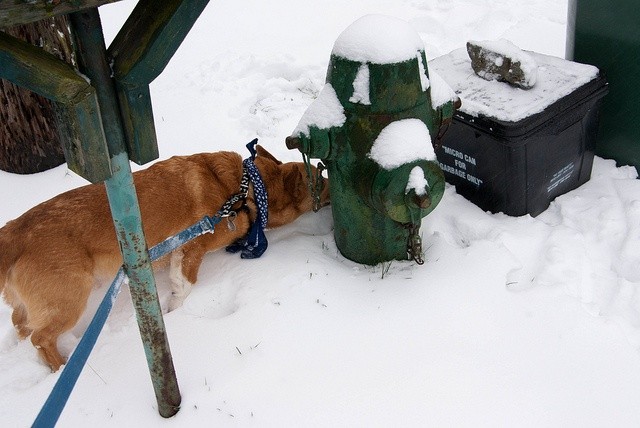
[425,41,609,218]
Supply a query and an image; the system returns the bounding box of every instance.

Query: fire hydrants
[284,14,463,267]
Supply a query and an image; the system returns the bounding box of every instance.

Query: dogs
[0,144,331,374]
